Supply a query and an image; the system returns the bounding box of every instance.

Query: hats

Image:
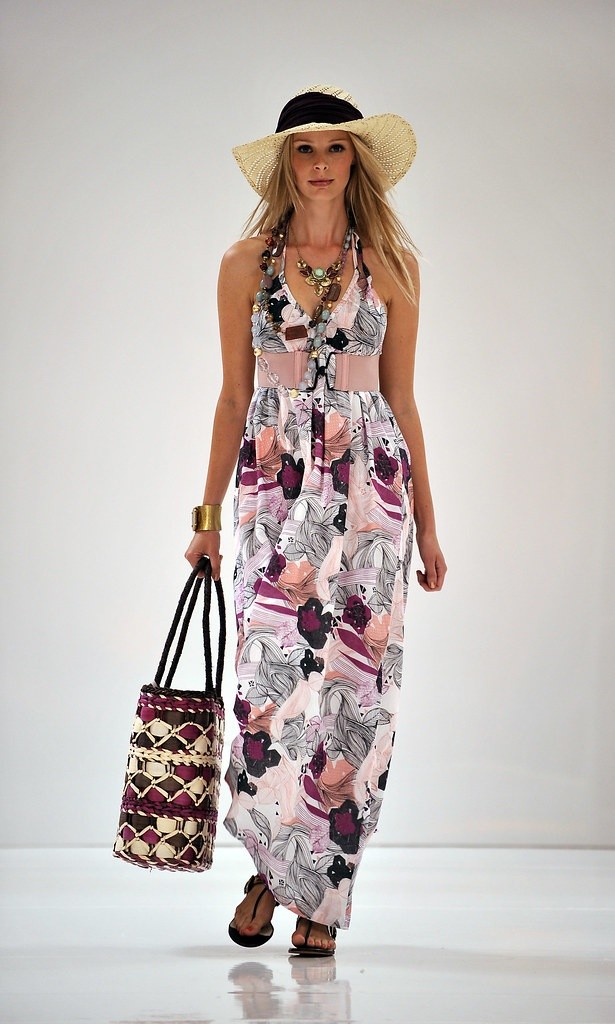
[232,84,418,204]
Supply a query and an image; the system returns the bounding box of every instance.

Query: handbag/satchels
[113,555,227,874]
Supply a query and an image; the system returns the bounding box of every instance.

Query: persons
[183,88,450,959]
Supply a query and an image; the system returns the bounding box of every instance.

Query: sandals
[228,875,280,949]
[289,913,336,955]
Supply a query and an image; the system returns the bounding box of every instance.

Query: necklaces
[252,222,353,399]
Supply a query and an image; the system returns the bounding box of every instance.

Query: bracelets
[188,506,223,535]
